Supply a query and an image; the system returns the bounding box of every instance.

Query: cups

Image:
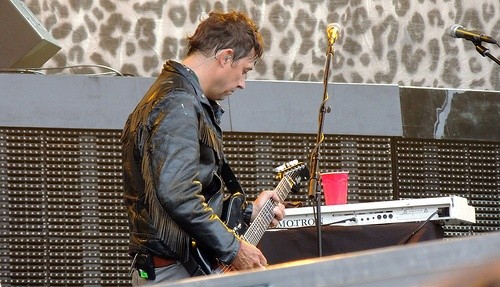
[321,171,349,205]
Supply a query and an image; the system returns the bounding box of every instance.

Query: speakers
[0,0,61,73]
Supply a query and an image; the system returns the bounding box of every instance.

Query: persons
[120,10,285,287]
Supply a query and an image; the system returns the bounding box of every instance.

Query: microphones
[447,24,497,44]
[326,22,342,58]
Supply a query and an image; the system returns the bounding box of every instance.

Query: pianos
[248,195,479,233]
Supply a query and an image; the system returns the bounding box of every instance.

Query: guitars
[211,158,311,276]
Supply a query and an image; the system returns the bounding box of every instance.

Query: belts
[153,255,178,268]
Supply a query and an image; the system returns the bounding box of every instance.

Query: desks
[257,221,445,266]
